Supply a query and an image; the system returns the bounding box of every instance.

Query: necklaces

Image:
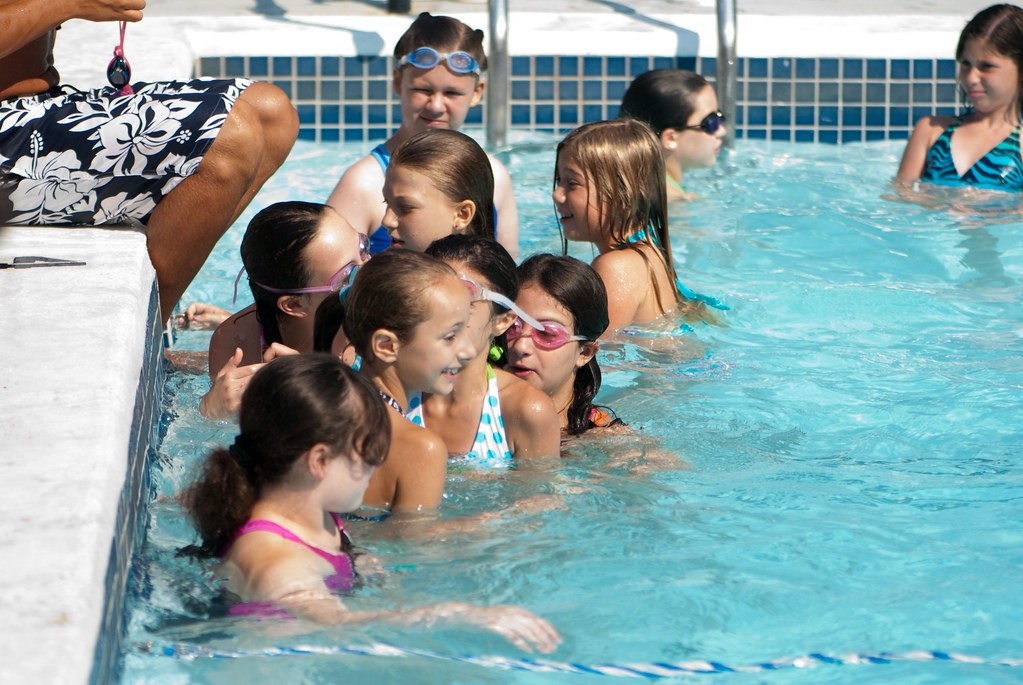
[380,392,403,415]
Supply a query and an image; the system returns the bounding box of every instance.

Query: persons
[311,250,472,518]
[550,118,674,339]
[325,12,518,259]
[617,69,726,196]
[403,235,559,463]
[381,129,493,253]
[1,0,298,321]
[896,4,1023,186]
[189,353,389,602]
[199,201,372,421]
[503,252,623,437]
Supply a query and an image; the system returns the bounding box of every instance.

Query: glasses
[278,232,370,295]
[397,47,483,75]
[456,277,486,301]
[504,314,596,350]
[108,53,131,99]
[657,110,726,139]
